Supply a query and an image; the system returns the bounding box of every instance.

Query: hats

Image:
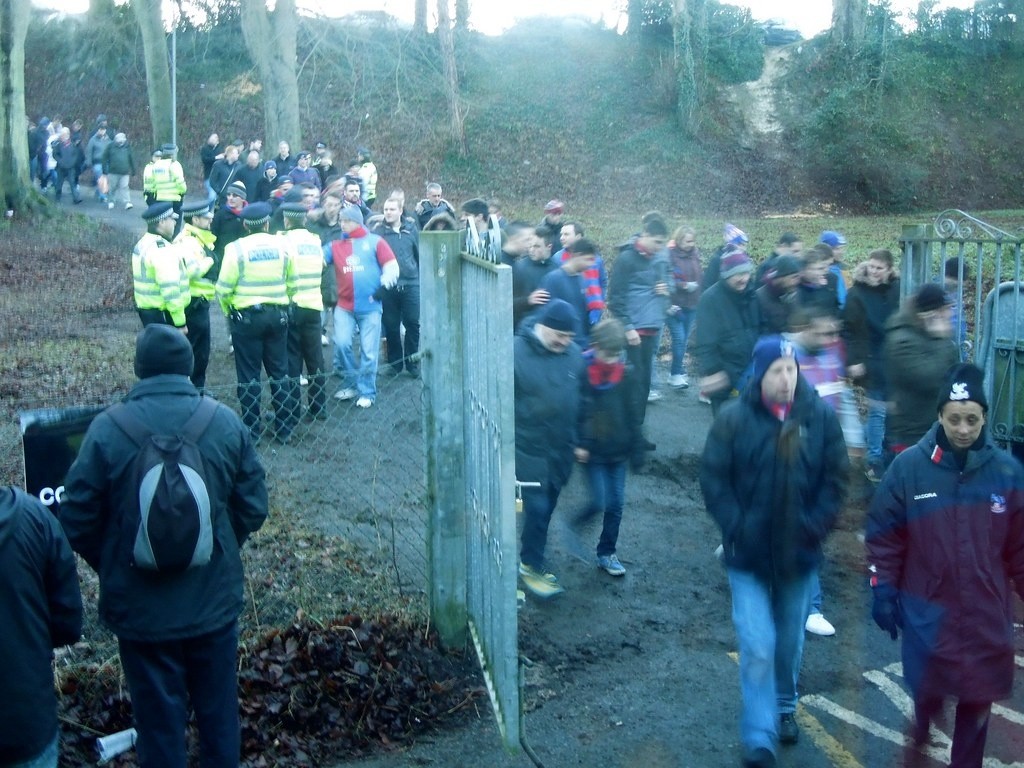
[240,201,273,225]
[233,138,244,146]
[935,361,988,411]
[296,153,307,159]
[462,198,489,222]
[180,200,214,218]
[752,333,796,384]
[545,199,564,214]
[277,175,293,185]
[265,161,278,169]
[915,282,950,313]
[536,298,577,332]
[339,205,364,224]
[141,201,179,223]
[225,180,247,200]
[718,225,754,279]
[316,141,326,148]
[134,322,194,378]
[162,144,178,154]
[282,201,308,218]
[776,255,805,276]
[821,231,847,247]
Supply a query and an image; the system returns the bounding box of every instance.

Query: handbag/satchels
[97,174,109,194]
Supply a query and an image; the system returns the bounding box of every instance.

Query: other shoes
[518,561,564,598]
[334,389,354,400]
[744,747,773,768]
[386,365,420,378]
[322,336,329,345]
[356,396,371,408]
[666,373,689,389]
[124,202,133,210]
[806,614,835,635]
[775,712,799,744]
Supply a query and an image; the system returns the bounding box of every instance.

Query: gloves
[869,584,906,641]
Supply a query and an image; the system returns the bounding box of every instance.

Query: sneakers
[598,554,626,575]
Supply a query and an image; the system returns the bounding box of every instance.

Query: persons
[132,132,970,635]
[0,485,82,768]
[864,362,1023,768]
[26,114,136,209]
[704,333,851,768]
[59,322,271,768]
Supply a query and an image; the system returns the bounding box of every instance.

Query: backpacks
[107,395,220,570]
